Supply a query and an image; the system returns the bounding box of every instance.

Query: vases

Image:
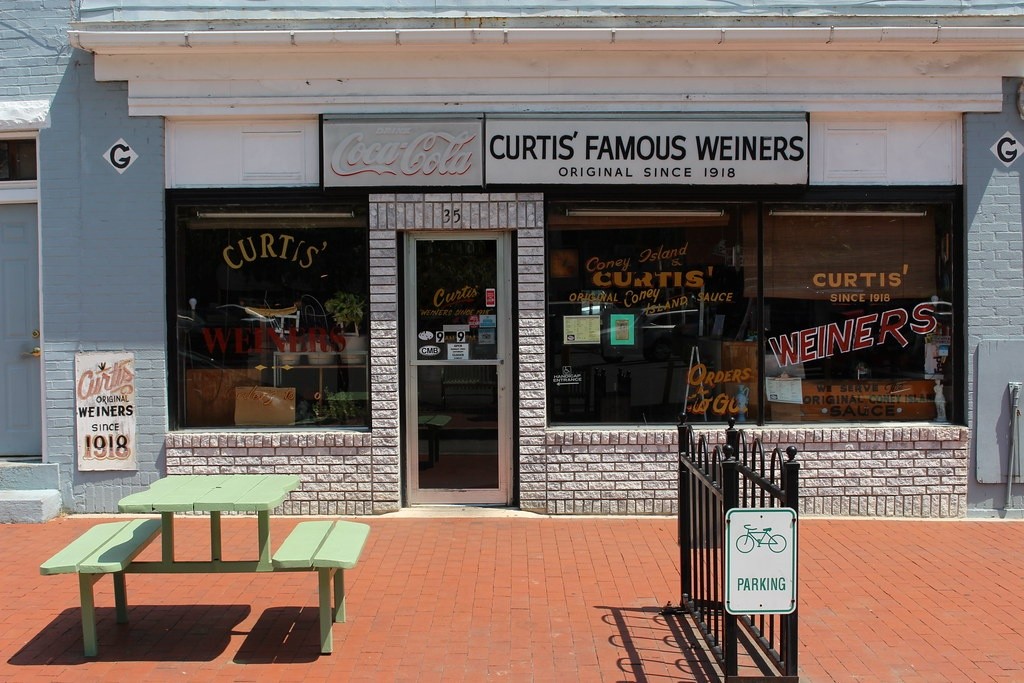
[278,342,302,366]
[305,334,335,365]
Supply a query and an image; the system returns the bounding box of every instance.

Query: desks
[117,475,318,573]
[325,392,369,425]
[722,339,758,419]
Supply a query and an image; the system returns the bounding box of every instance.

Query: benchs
[288,415,329,425]
[270,520,371,653]
[440,356,498,410]
[39,518,161,657]
[418,415,453,468]
[549,362,593,416]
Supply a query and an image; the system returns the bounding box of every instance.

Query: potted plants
[325,291,368,364]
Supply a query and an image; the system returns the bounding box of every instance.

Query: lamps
[768,207,928,217]
[564,206,726,217]
